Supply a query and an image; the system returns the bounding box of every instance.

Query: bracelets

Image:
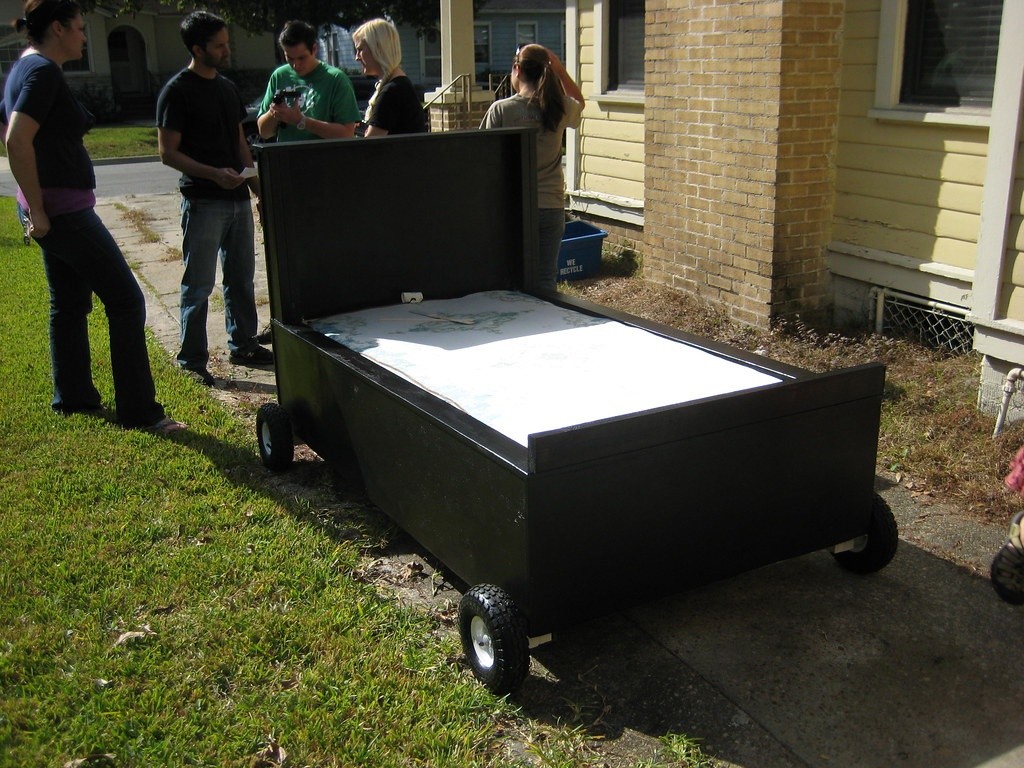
[255,192,262,200]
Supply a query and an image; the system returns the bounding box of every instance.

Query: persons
[256,21,362,142]
[156,12,274,386]
[991,448,1024,606]
[0,0,188,432]
[479,44,584,292]
[352,19,427,137]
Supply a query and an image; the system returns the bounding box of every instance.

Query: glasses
[516,42,534,63]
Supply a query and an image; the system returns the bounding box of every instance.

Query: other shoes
[143,416,189,435]
[86,403,108,417]
[182,366,216,387]
[228,345,273,365]
[989,510,1024,605]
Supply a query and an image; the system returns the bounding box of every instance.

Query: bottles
[284,86,295,109]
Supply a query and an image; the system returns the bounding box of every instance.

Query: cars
[240,94,280,152]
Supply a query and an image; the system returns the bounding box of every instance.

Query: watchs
[297,116,306,130]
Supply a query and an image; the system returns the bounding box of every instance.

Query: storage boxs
[556,220,608,283]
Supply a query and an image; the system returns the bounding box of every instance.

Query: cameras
[272,85,300,108]
